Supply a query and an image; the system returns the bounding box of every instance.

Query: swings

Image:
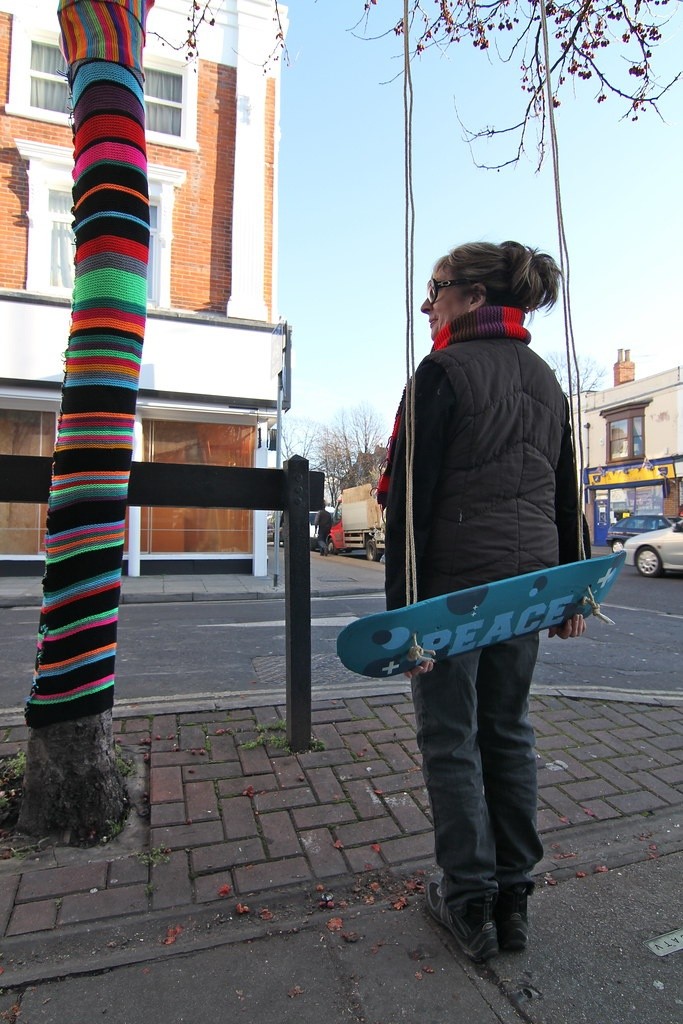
[332,1,629,680]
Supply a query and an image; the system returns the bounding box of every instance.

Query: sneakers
[498,884,529,952]
[426,880,502,962]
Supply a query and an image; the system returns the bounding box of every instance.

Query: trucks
[267,496,386,561]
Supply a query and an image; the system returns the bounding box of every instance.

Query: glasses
[425,278,477,305]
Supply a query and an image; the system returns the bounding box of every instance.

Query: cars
[606,515,683,577]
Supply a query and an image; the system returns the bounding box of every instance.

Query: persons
[314,506,333,557]
[370,241,591,955]
